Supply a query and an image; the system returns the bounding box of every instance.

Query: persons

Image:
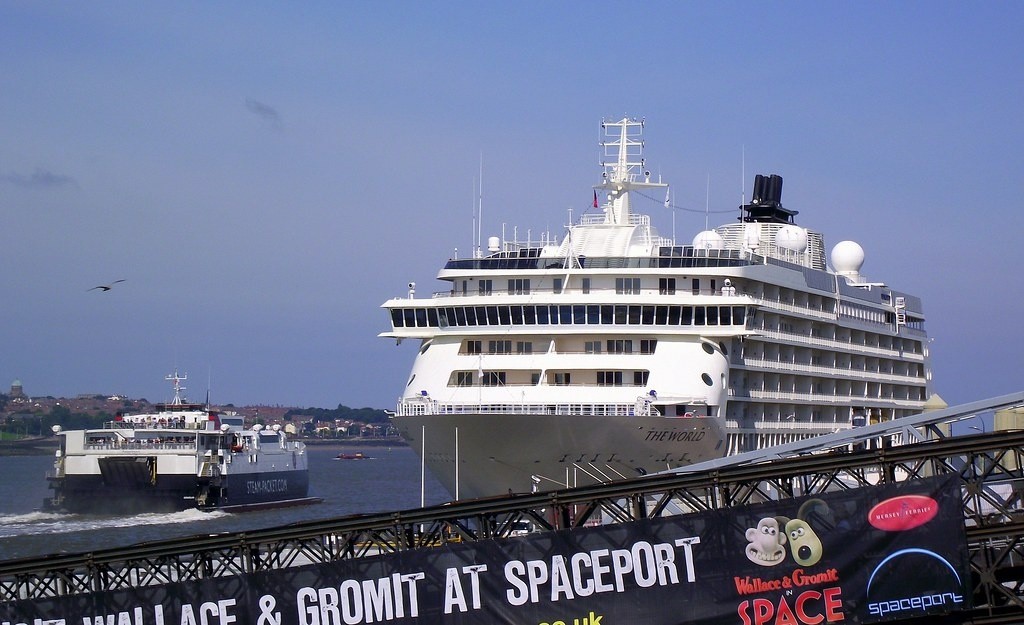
[92,416,196,449]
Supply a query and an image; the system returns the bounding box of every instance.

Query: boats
[335,451,370,459]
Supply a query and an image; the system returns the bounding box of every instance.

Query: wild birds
[84,277,126,293]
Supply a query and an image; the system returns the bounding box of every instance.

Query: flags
[664,187,670,208]
[594,189,598,208]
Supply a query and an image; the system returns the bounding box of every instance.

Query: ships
[44,365,307,507]
[375,113,935,525]
